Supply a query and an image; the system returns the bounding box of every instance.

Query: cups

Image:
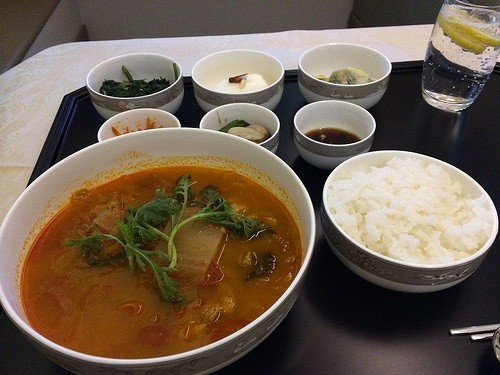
[421,0,500,114]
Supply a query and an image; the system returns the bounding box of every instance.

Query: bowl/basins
[199,102,280,154]
[320,150,499,293]
[293,99,376,171]
[297,42,392,110]
[85,52,184,120]
[96,108,181,142]
[191,48,285,112]
[0,127,317,375]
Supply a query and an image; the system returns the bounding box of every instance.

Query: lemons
[438,5,500,52]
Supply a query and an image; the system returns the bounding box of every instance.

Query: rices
[327,157,494,265]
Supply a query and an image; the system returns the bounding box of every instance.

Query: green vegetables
[61,173,272,303]
[100,62,178,97]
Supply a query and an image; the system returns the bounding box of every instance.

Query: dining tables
[0,25,500,375]
